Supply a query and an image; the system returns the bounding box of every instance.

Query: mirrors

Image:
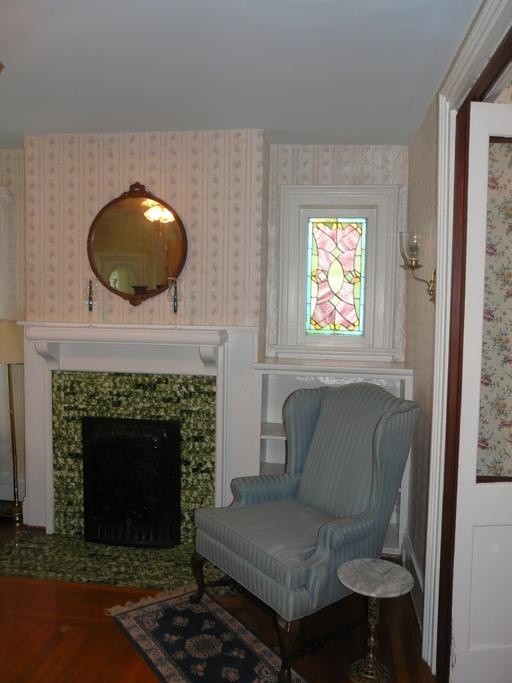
[94,249,146,295]
[86,181,188,306]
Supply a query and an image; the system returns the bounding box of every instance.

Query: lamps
[139,196,174,224]
[400,229,436,305]
[0,314,24,524]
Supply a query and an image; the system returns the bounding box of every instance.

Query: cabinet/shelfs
[259,360,415,554]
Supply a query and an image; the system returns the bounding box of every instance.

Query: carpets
[107,582,313,682]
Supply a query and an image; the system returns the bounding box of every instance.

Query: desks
[336,558,415,681]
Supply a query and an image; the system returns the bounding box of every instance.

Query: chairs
[188,380,420,682]
